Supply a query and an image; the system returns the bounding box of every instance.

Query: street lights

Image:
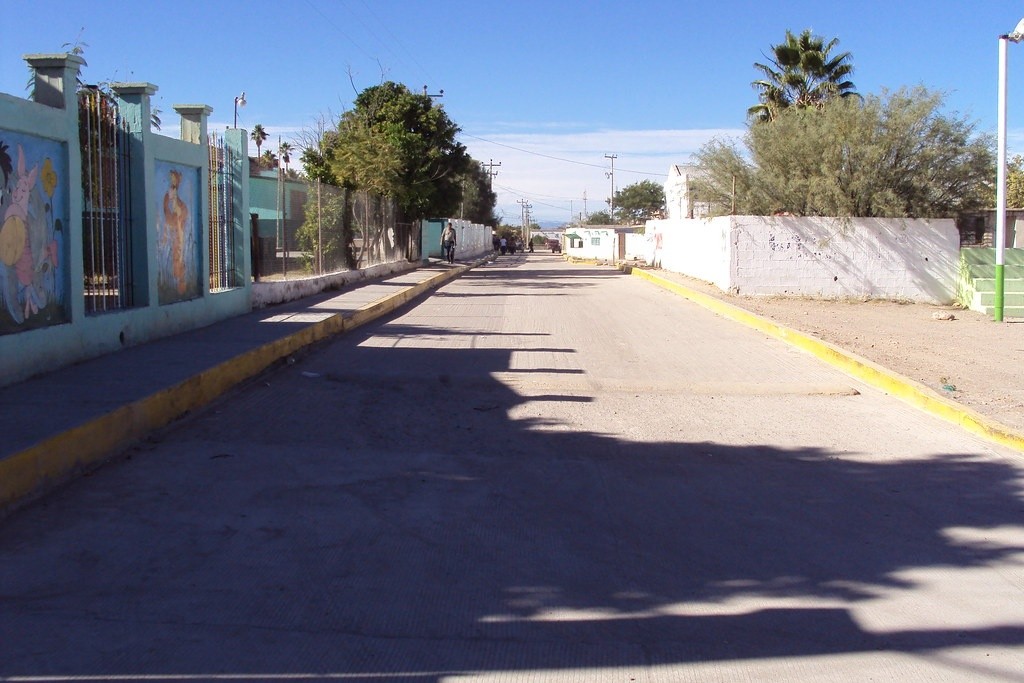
[234,96,246,128]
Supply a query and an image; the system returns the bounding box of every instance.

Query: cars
[551,242,562,253]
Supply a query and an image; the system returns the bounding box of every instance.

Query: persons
[492,233,535,255]
[439,223,457,264]
